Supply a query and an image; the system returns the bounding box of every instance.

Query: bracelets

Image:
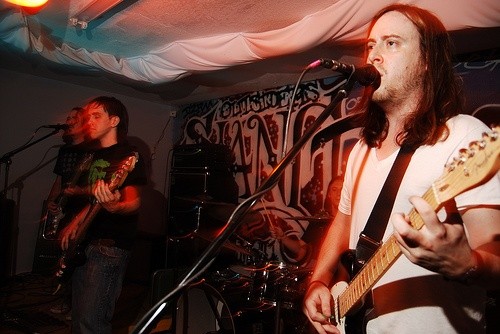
[468,249,483,276]
[307,280,329,290]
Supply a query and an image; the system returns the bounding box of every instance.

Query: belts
[90,238,115,248]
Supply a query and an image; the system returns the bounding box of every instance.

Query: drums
[176,281,253,334]
[256,260,306,310]
[228,263,257,278]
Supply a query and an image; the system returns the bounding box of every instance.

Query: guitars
[50,151,140,296]
[300,127,500,334]
[40,153,94,241]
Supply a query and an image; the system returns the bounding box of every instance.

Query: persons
[48,106,89,321]
[61,95,143,334]
[304,3,500,334]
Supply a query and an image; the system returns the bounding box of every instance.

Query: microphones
[321,58,378,86]
[44,124,69,130]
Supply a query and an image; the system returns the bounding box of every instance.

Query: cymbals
[173,195,235,206]
[284,216,332,221]
[240,266,263,271]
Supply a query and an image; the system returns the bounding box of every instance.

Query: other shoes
[65,310,72,320]
[49,303,70,313]
